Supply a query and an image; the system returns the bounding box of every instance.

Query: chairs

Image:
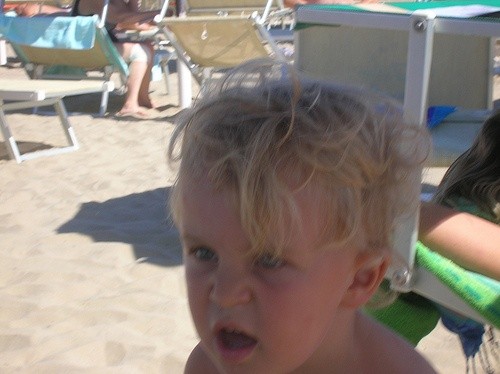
[0,11,291,163]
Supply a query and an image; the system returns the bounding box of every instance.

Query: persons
[0,3,72,17]
[417,109,500,282]
[72,0,174,120]
[167,56,440,374]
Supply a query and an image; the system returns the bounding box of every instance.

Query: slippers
[115,106,158,120]
